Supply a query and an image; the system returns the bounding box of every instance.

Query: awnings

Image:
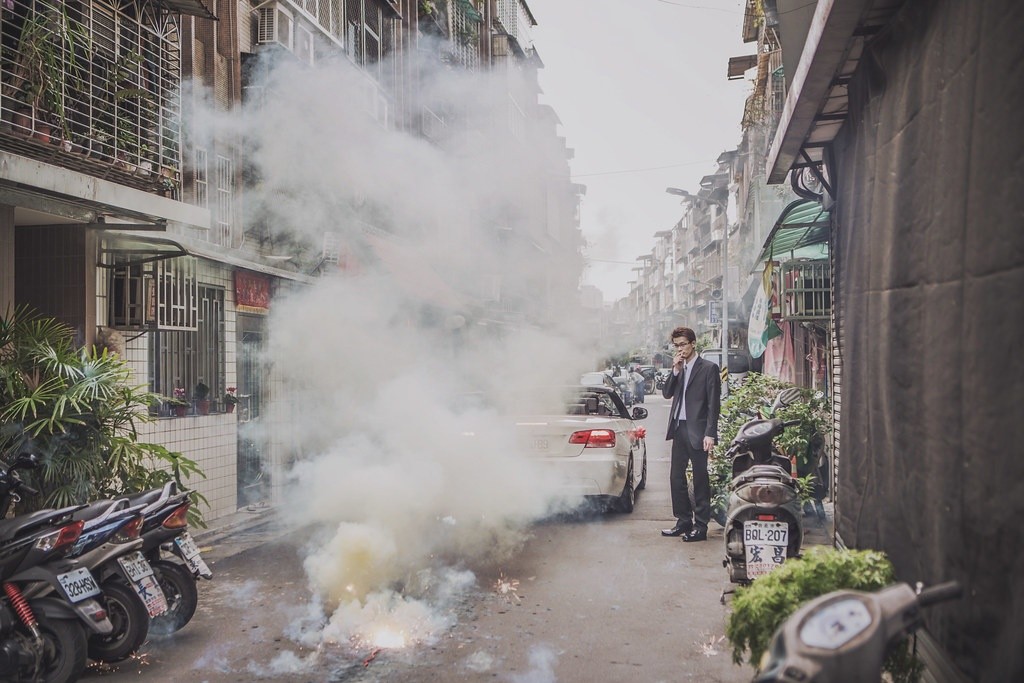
[96,230,323,288]
[749,197,830,274]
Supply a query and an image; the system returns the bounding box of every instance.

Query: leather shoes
[683,530,706,542]
[662,525,692,536]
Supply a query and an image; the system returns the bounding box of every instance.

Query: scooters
[752,580,965,683]
[720,387,802,606]
[0,453,214,683]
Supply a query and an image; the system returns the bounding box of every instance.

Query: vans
[701,349,753,394]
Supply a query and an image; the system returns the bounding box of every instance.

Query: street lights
[665,187,727,400]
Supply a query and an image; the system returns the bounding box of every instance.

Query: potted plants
[8,9,177,187]
[193,383,212,415]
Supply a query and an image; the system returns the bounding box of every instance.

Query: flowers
[163,387,189,404]
[224,387,240,404]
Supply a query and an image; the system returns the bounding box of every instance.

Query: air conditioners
[797,274,834,315]
[112,277,164,323]
[257,2,294,53]
[295,23,315,66]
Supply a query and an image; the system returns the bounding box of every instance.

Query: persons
[611,364,621,377]
[628,364,644,377]
[662,327,721,542]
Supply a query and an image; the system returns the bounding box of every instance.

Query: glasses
[673,341,692,349]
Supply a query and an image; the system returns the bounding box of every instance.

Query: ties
[675,366,687,431]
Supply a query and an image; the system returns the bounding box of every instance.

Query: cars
[522,355,674,513]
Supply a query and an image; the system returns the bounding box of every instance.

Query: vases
[176,404,187,418]
[226,405,236,412]
